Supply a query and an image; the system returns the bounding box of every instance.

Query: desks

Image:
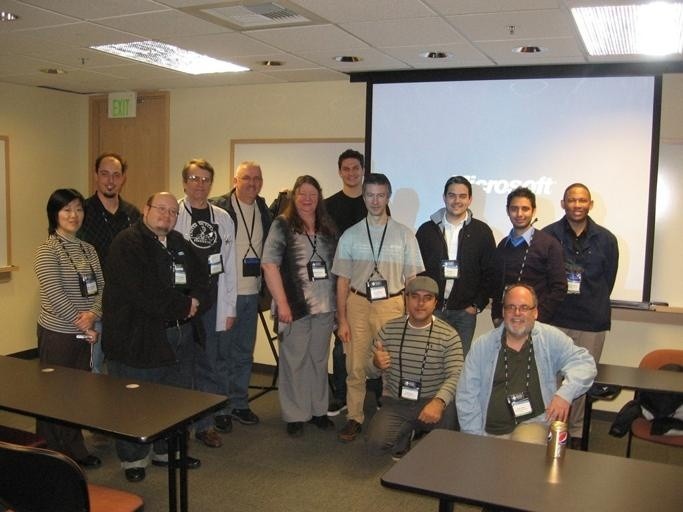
[380,429,683,512]
[579,364,683,452]
[0,355,230,512]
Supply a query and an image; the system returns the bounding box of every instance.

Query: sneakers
[76,454,103,468]
[194,426,223,448]
[309,414,335,432]
[286,421,305,436]
[124,466,146,483]
[326,400,348,417]
[232,408,260,424]
[338,419,362,441]
[151,453,200,469]
[215,414,234,433]
[391,428,416,461]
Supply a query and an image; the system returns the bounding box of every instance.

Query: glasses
[504,305,537,313]
[151,204,178,216]
[236,175,261,182]
[186,175,210,183]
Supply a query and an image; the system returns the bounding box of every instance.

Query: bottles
[546,420,569,459]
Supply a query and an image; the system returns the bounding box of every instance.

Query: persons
[173,159,237,450]
[74,153,140,456]
[101,191,216,481]
[491,187,568,330]
[455,283,598,444]
[36,188,104,468]
[208,161,272,434]
[361,275,464,465]
[322,149,390,417]
[331,173,426,442]
[261,175,338,439]
[415,176,497,362]
[541,183,619,450]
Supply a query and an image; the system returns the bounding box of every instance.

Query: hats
[405,275,439,297]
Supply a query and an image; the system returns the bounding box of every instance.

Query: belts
[162,315,193,330]
[350,286,402,301]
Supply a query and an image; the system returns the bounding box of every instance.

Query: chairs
[610,350,683,457]
[1,442,144,512]
